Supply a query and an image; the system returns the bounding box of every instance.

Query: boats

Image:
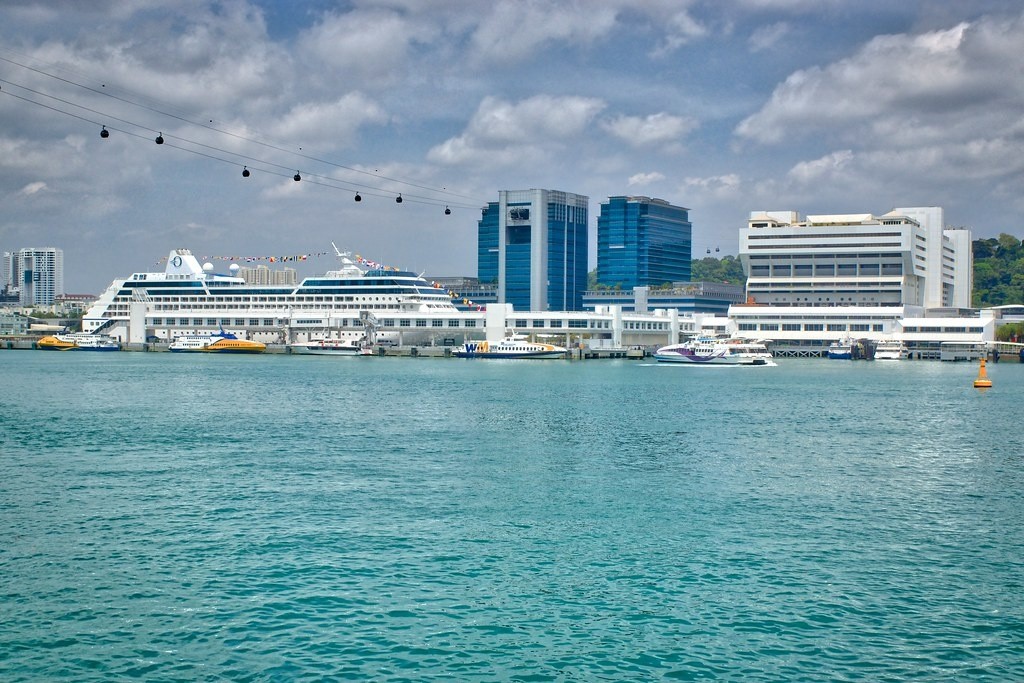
[873,339,908,359]
[290,335,372,355]
[167,321,267,354]
[828,334,867,360]
[451,329,568,359]
[36,331,123,351]
[653,328,775,365]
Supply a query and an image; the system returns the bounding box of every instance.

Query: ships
[79,240,460,345]
